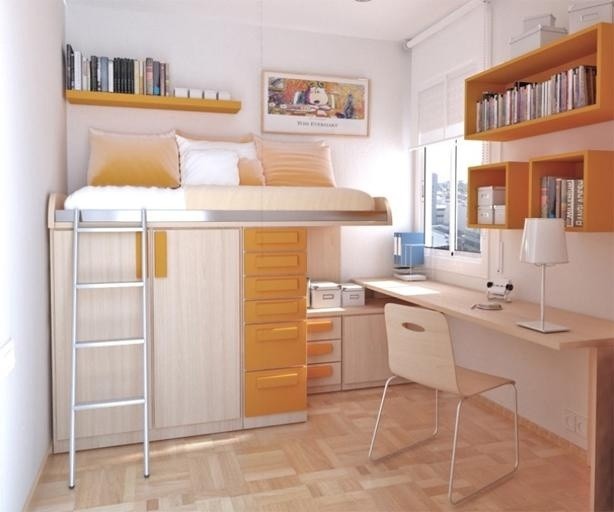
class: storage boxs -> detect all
[523,14,556,32]
[339,282,365,307]
[567,0,614,35]
[510,23,568,58]
[310,281,342,309]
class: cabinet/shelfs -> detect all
[528,149,614,232]
[464,22,614,141]
[467,161,528,229]
[307,316,342,394]
[49,226,308,453]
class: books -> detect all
[475,63,597,133]
[540,175,583,228]
[65,42,171,96]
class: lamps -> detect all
[517,217,571,333]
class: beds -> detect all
[47,186,392,228]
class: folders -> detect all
[393,232,425,266]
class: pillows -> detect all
[176,130,265,187]
[254,137,326,147]
[261,144,336,187]
[86,127,180,188]
[176,130,255,143]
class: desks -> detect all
[352,278,614,512]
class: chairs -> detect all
[367,302,519,506]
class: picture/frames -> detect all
[261,68,370,137]
